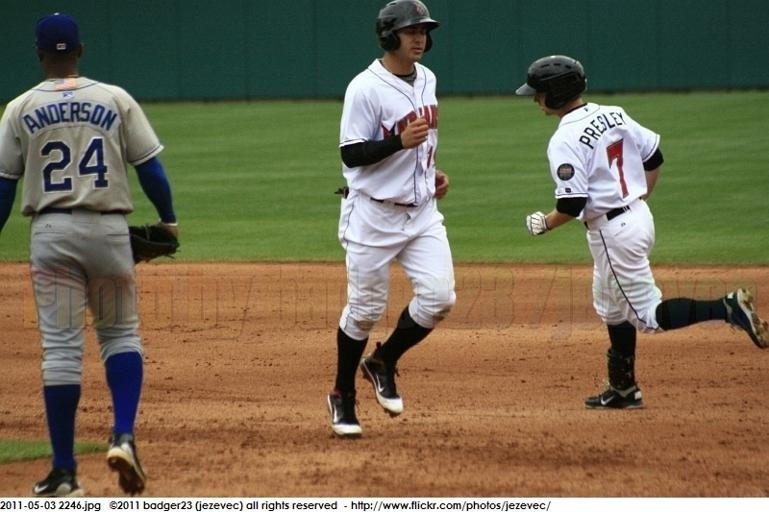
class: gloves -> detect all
[526,211,549,238]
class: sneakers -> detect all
[361,355,407,418]
[326,389,365,438]
[723,287,768,351]
[582,387,645,412]
[106,436,147,497]
[31,472,82,498]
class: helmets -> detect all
[516,56,586,111]
[376,0,440,51]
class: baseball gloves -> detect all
[129,225,178,264]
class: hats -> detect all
[34,11,80,52]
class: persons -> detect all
[322,1,462,441]
[512,52,768,412]
[0,11,183,497]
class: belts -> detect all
[584,195,643,230]
[38,208,124,215]
